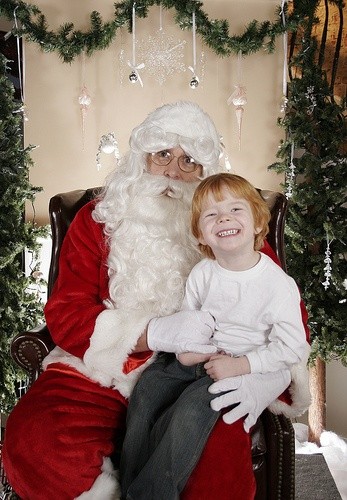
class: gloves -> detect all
[207,366,292,433]
[147,310,218,353]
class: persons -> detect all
[120,174,306,499]
[2,102,312,499]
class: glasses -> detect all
[149,149,200,172]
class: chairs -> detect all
[9,185,295,500]
